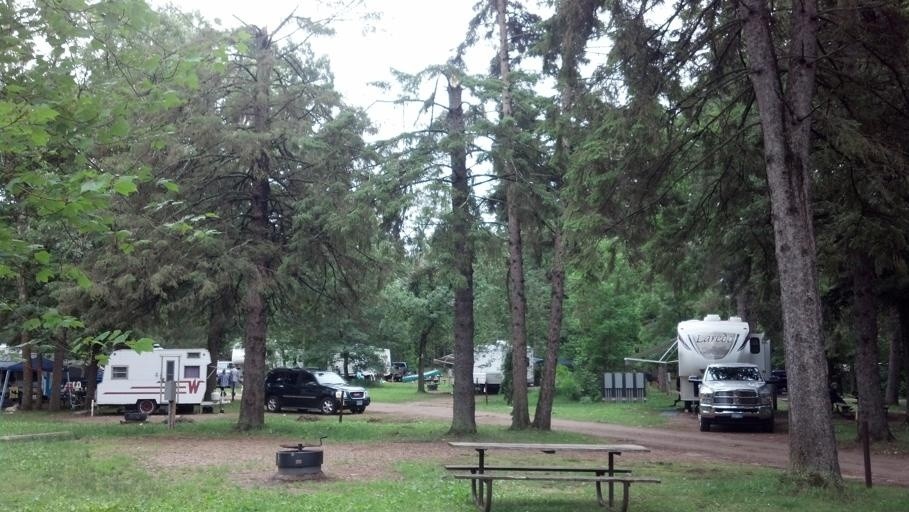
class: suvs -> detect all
[216,360,240,387]
[264,367,371,415]
[385,361,412,382]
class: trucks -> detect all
[448,360,506,394]
[687,363,780,433]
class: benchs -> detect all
[445,462,631,501]
[454,472,662,510]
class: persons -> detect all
[217,367,229,403]
[229,365,240,402]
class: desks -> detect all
[447,441,651,511]
